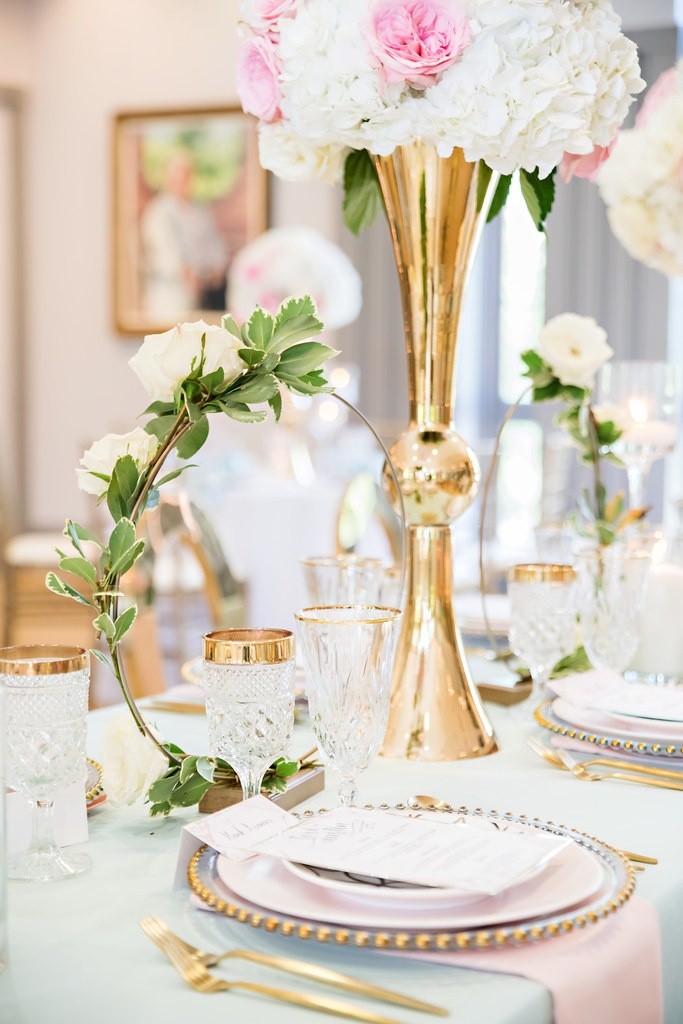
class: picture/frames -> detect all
[107,94,270,332]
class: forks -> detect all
[559,750,683,791]
[142,913,447,1015]
[526,736,682,779]
[154,936,402,1024]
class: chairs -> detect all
[178,491,250,629]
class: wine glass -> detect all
[201,628,296,801]
[1,645,90,880]
[509,563,579,722]
[294,605,403,809]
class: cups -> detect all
[305,556,381,605]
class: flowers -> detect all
[596,59,683,279]
[44,297,343,813]
[235,0,647,182]
[519,308,650,675]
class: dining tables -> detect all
[0,554,683,1024]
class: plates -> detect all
[278,847,488,911]
[85,757,104,802]
[553,696,682,742]
[188,804,635,948]
[534,698,683,757]
[213,811,605,930]
